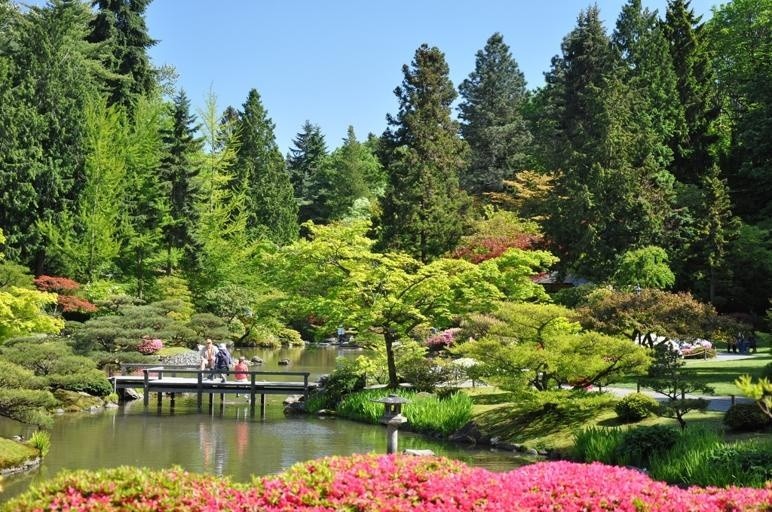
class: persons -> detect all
[199,339,220,381]
[202,342,234,382]
[233,356,249,399]
